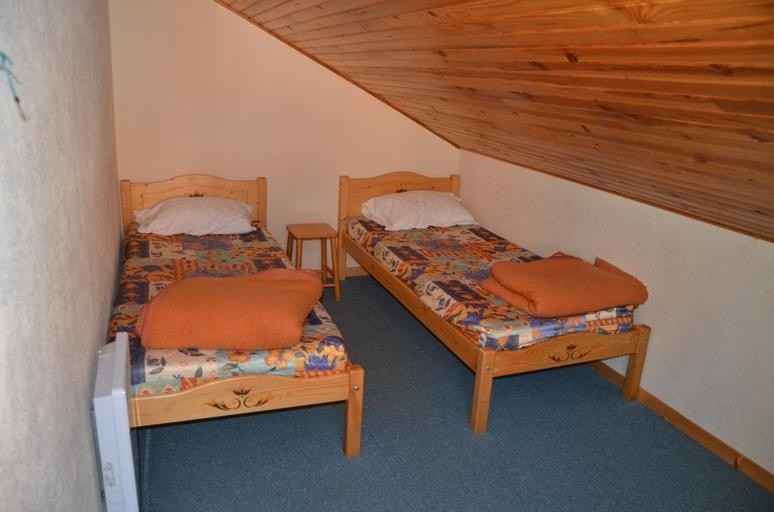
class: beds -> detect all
[103,170,366,458]
[333,168,654,433]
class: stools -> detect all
[284,222,341,303]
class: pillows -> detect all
[129,194,262,239]
[356,189,481,234]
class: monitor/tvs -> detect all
[93,331,142,512]
